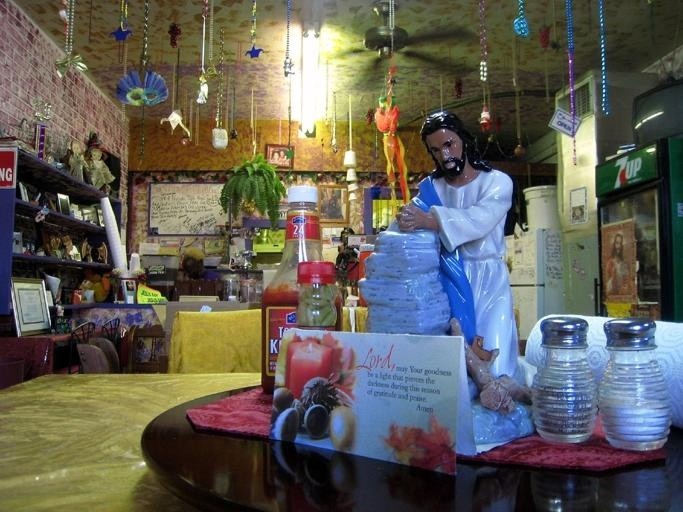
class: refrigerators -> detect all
[594,137,683,321]
[505,230,543,353]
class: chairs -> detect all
[88,337,120,374]
[54,322,96,374]
[167,308,263,373]
[76,344,110,374]
[99,317,120,343]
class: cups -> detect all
[99,195,142,274]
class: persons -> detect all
[271,149,288,162]
[396,110,526,385]
[66,139,117,191]
[604,232,633,296]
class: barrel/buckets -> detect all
[522,185,562,232]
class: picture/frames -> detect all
[266,144,295,169]
[10,276,52,338]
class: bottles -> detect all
[240,251,257,271]
[260,183,340,396]
[222,274,257,304]
[530,314,673,452]
[526,464,670,509]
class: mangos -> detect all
[329,405,354,450]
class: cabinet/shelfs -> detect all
[172,280,227,302]
[0,146,121,336]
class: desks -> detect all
[0,373,682,512]
